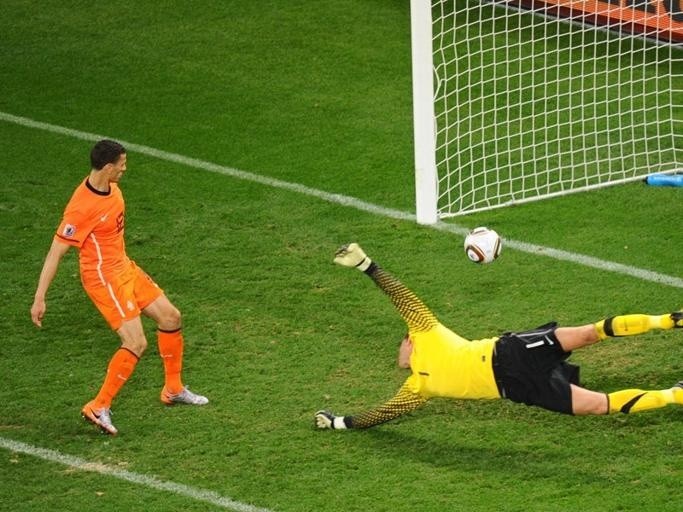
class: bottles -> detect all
[642,174,683,186]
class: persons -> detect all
[308,241,682,431]
[30,138,210,437]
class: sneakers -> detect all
[160,384,209,406]
[80,400,118,434]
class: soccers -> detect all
[464,227,503,264]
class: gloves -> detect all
[332,242,372,272]
[315,411,337,430]
